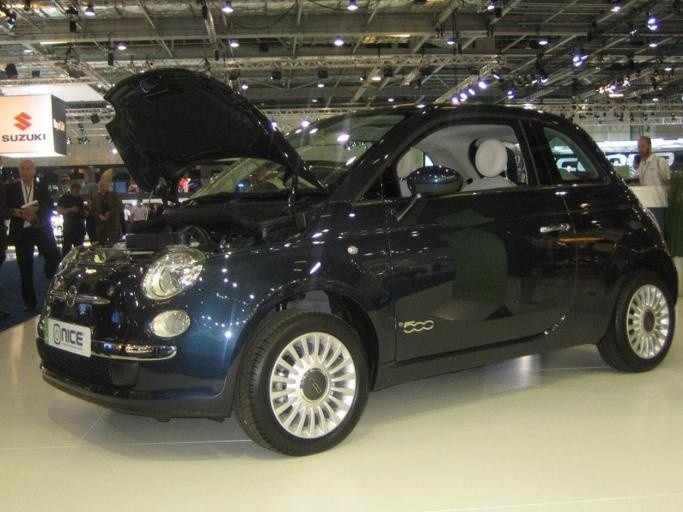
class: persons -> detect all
[96,182,123,243]
[124,204,131,222]
[131,199,146,224]
[633,155,641,174]
[57,184,86,256]
[637,136,671,239]
[0,160,59,312]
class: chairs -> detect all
[462,140,518,191]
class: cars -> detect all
[35,66,679,458]
